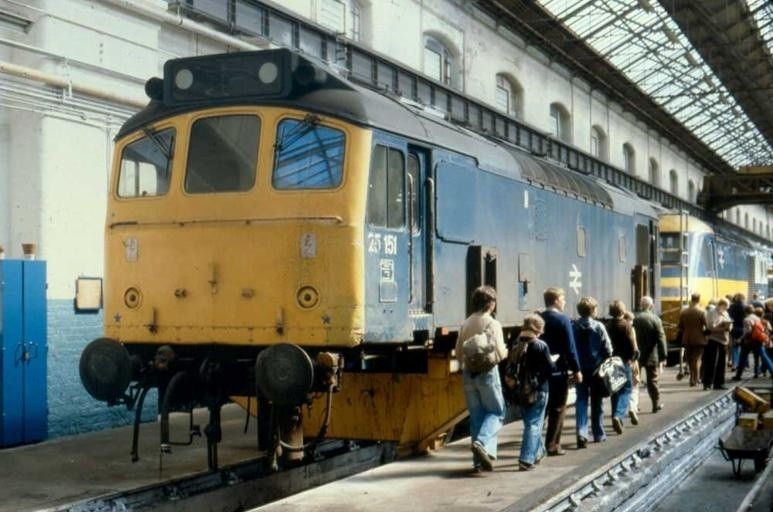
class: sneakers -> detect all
[469,404,664,473]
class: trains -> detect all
[78,43,772,480]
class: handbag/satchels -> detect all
[462,330,500,379]
[592,356,628,397]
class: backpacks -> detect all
[752,319,769,345]
[502,338,545,408]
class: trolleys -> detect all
[712,382,771,479]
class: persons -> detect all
[572,297,614,449]
[538,287,584,456]
[455,285,509,474]
[624,313,639,426]
[604,300,633,434]
[633,296,668,412]
[675,291,773,392]
[499,314,554,472]
